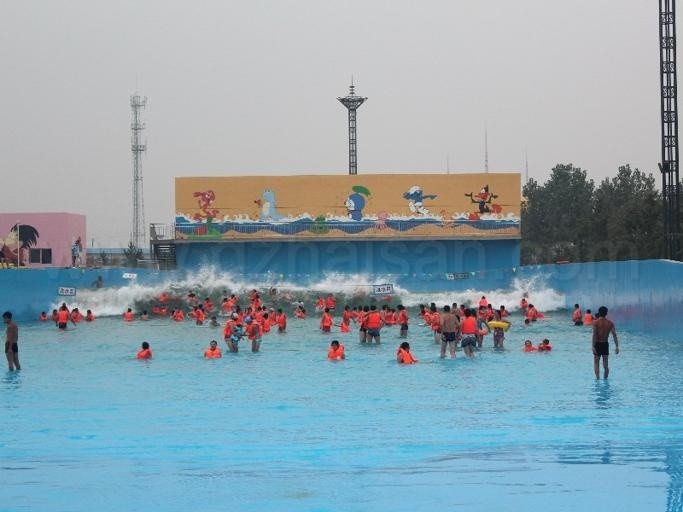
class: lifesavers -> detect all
[478,321,508,335]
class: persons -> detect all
[3,312,21,370]
[39,288,599,365]
[593,306,619,379]
